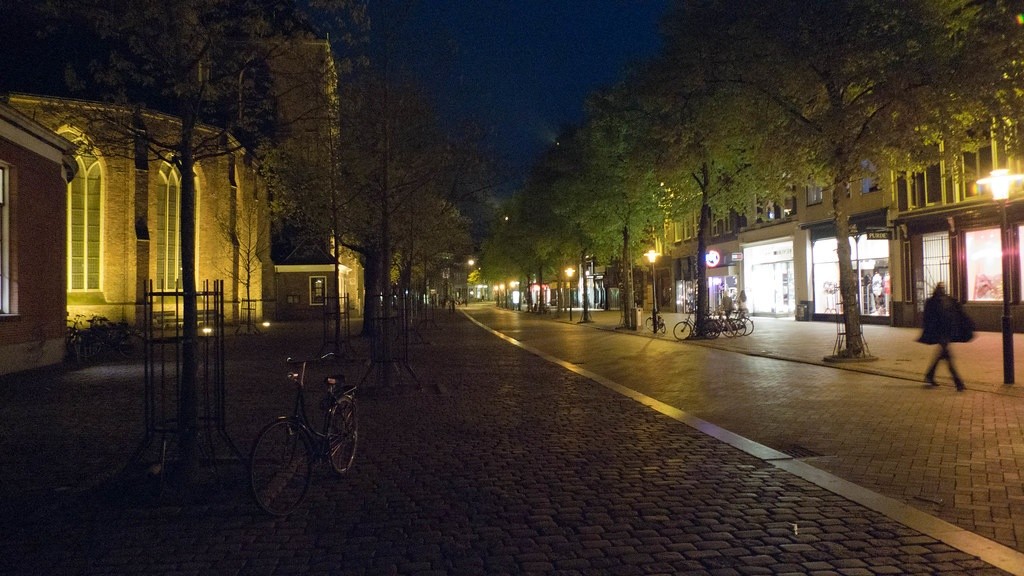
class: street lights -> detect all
[564,268,575,321]
[643,249,661,334]
[975,167,1024,384]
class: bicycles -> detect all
[247,352,360,518]
[62,314,145,359]
[646,308,666,334]
[673,308,755,340]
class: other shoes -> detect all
[925,376,939,387]
[954,378,966,391]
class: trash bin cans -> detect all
[630,306,644,330]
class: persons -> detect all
[866,271,890,296]
[723,292,732,310]
[736,290,747,309]
[914,282,977,392]
